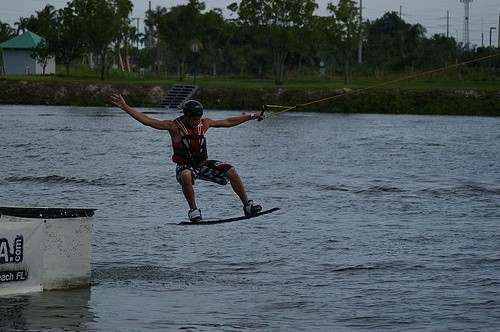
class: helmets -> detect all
[184,101,203,116]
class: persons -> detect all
[110,91,265,222]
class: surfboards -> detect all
[178,207,280,225]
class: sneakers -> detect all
[243,200,261,214]
[188,209,202,220]
